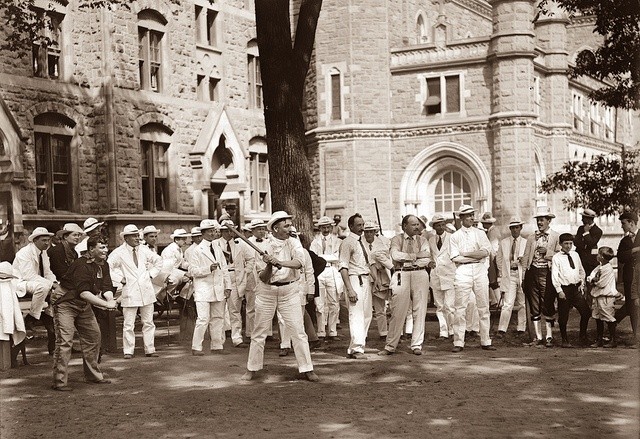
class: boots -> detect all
[589,319,604,348]
[560,324,572,348]
[579,322,593,345]
[603,322,617,348]
[10,338,23,369]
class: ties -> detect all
[437,236,442,250]
[406,238,414,255]
[39,251,43,278]
[512,238,516,261]
[564,252,575,269]
[226,241,233,264]
[369,244,372,252]
[209,243,216,261]
[465,229,473,252]
[357,236,369,264]
[133,248,138,268]
[322,237,326,253]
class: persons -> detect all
[361,222,389,340]
[418,214,434,240]
[160,229,194,306]
[377,206,431,356]
[479,211,502,310]
[141,224,166,307]
[51,234,118,391]
[496,215,527,339]
[107,224,162,355]
[338,214,373,360]
[464,290,480,337]
[10,224,54,325]
[75,215,108,257]
[322,286,343,330]
[449,205,493,352]
[310,216,344,343]
[400,298,412,341]
[428,214,457,340]
[216,220,255,347]
[611,212,640,342]
[47,222,83,281]
[587,247,618,347]
[241,212,319,381]
[551,232,591,346]
[575,209,603,274]
[182,219,229,356]
[522,207,557,346]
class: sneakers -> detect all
[71,346,81,354]
[49,350,54,355]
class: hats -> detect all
[314,216,335,227]
[479,212,496,224]
[141,225,161,239]
[82,217,104,233]
[247,219,270,229]
[240,223,252,232]
[56,222,87,238]
[195,219,218,231]
[454,205,478,216]
[429,214,449,227]
[267,211,296,232]
[363,221,380,231]
[333,215,341,221]
[532,206,556,218]
[169,228,190,240]
[579,209,598,217]
[218,220,238,229]
[120,224,142,241]
[289,225,301,235]
[211,219,221,230]
[190,226,202,236]
[506,216,525,227]
[416,215,428,229]
[28,227,55,242]
[474,215,481,223]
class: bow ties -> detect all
[535,231,550,242]
[148,245,154,249]
[256,238,263,242]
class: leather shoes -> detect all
[51,383,73,391]
[377,349,394,355]
[436,336,448,341]
[464,330,470,340]
[123,354,134,359]
[328,336,341,341]
[482,345,497,351]
[226,330,231,337]
[349,352,367,359]
[236,343,249,348]
[451,334,454,342]
[40,314,53,333]
[278,348,290,356]
[528,337,543,346]
[319,337,326,341]
[23,315,37,332]
[211,349,231,355]
[245,337,251,344]
[240,369,255,381]
[413,348,422,355]
[451,346,463,353]
[266,336,277,342]
[146,353,159,357]
[470,331,478,337]
[545,337,554,346]
[400,335,406,340]
[496,331,504,339]
[406,333,412,340]
[380,336,386,339]
[305,371,319,382]
[174,296,187,306]
[518,331,525,339]
[94,379,112,384]
[309,340,321,351]
[192,350,204,356]
[336,324,342,329]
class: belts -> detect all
[268,277,301,286]
[227,269,234,272]
[395,267,425,271]
[510,266,518,270]
[563,283,581,287]
[348,273,369,285]
[460,262,481,264]
